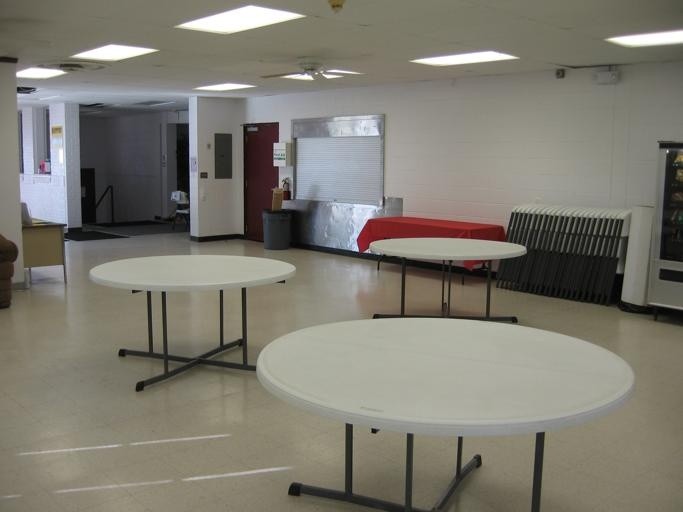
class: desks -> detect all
[89,254,296,391]
[256,318,635,512]
[358,217,505,285]
[369,238,527,322]
[23,219,69,284]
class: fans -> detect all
[261,63,362,80]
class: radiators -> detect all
[494,204,633,308]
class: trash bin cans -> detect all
[262,209,291,250]
